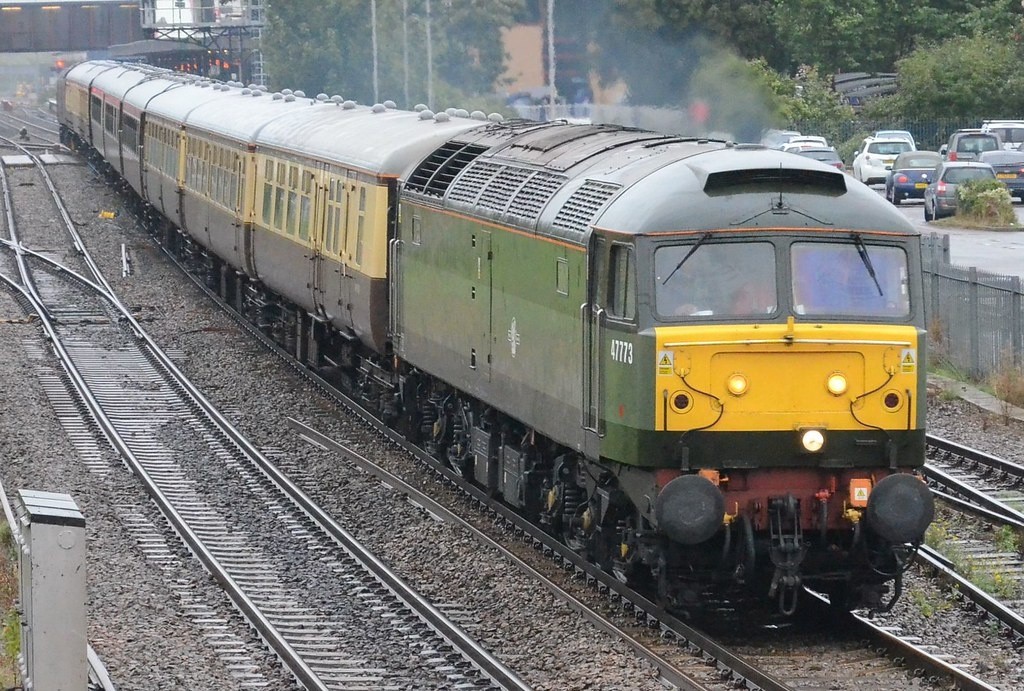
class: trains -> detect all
[52,34,935,639]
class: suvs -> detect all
[850,136,914,187]
[981,117,1024,150]
[945,128,1004,163]
[921,161,996,222]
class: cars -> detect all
[873,129,920,149]
[938,143,947,159]
[885,150,944,205]
[766,129,847,176]
[974,150,1024,205]
